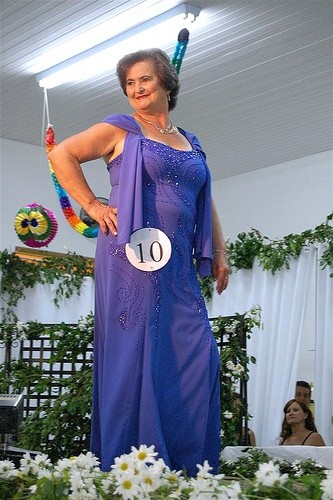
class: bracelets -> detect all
[212,250,227,256]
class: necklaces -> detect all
[144,119,174,134]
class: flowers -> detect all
[0,444,325,500]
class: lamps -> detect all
[33,2,202,89]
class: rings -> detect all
[225,277,228,280]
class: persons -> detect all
[224,392,256,447]
[48,49,230,479]
[277,380,326,446]
